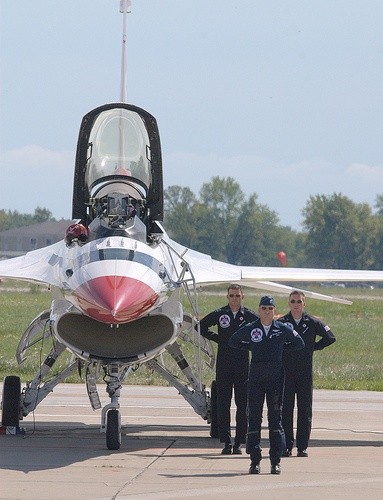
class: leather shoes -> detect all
[282,448,293,457]
[270,463,281,473]
[249,461,261,474]
[222,445,232,454]
[233,446,242,454]
[296,449,309,456]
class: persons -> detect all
[194,283,260,455]
[277,290,336,457]
[229,296,305,474]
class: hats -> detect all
[259,295,275,307]
[65,223,89,238]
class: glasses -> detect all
[261,307,273,311]
[290,300,302,304]
[228,294,241,298]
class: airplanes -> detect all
[0,95,383,453]
